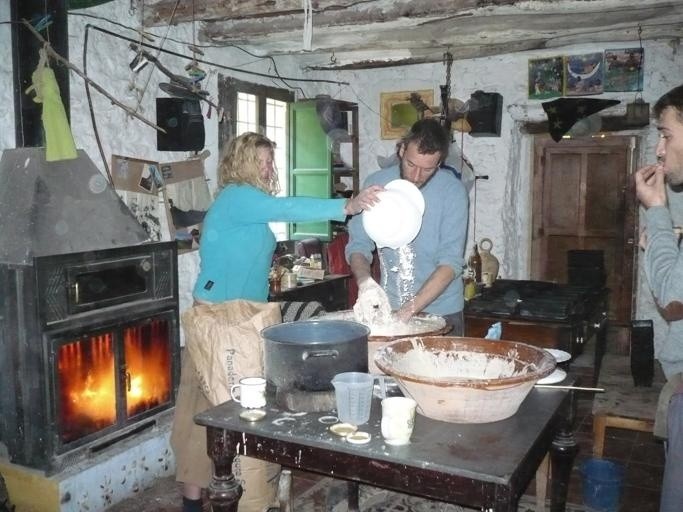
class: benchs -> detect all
[591,325,667,460]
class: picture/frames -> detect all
[381,88,434,140]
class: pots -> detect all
[258,317,371,414]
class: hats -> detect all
[159,75,206,101]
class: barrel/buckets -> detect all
[580,458,627,512]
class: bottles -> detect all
[469,240,481,281]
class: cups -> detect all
[267,274,281,296]
[283,271,298,291]
[310,253,323,270]
[380,395,417,446]
[229,376,268,409]
[329,370,386,428]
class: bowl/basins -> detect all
[328,305,452,371]
[362,179,425,251]
[376,337,556,425]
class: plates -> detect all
[537,365,567,385]
[297,278,316,284]
[545,347,572,363]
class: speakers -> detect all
[156,98,205,151]
[468,91,503,136]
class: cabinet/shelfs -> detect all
[297,96,360,236]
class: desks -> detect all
[269,273,351,312]
[195,371,584,512]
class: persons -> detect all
[342,116,469,337]
[632,82,682,380]
[169,132,386,512]
